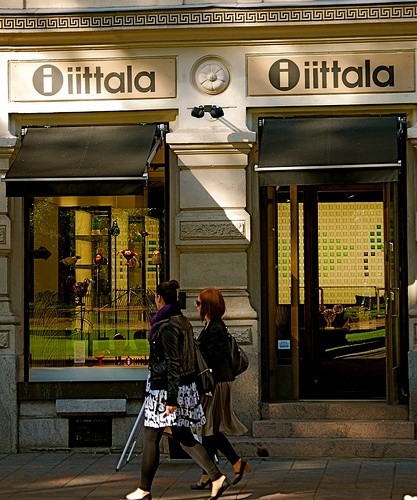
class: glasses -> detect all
[196,300,202,306]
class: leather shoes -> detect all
[208,476,232,500]
[119,492,152,500]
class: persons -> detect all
[189,287,251,490]
[120,279,232,500]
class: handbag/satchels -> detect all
[194,340,214,394]
[226,332,249,376]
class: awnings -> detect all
[0,125,162,179]
[256,112,406,187]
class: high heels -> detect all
[190,478,212,489]
[232,458,251,485]
